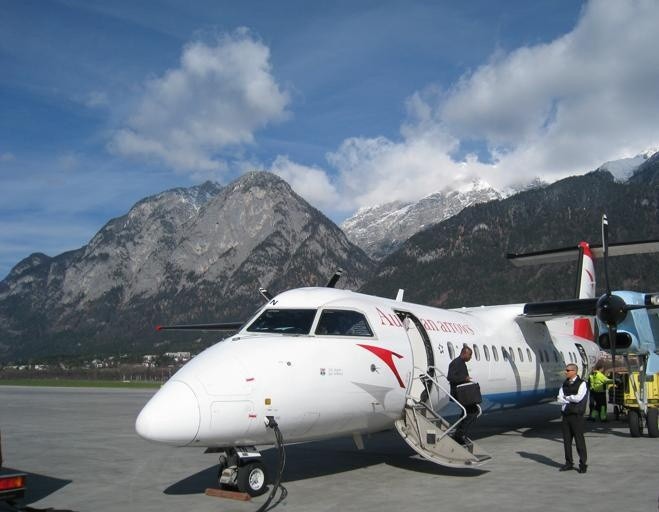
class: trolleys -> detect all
[605,370,639,420]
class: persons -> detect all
[557,364,588,473]
[589,365,613,423]
[446,346,479,445]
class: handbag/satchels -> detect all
[456,382,482,405]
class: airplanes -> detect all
[134,213,659,495]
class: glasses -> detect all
[566,369,575,372]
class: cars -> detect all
[0,465,25,507]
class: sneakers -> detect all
[560,466,574,471]
[578,467,587,474]
